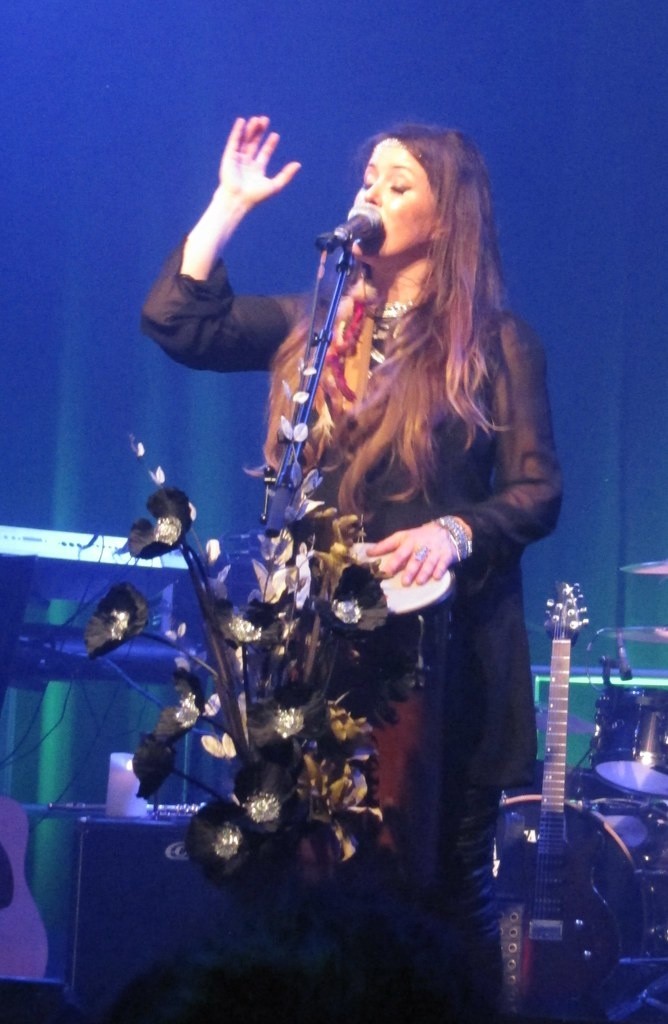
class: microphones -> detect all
[314,205,381,254]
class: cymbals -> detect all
[598,627,667,644]
[618,562,668,575]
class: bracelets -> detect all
[429,513,473,565]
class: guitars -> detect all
[499,584,625,1022]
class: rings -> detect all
[412,547,432,561]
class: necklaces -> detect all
[371,298,414,314]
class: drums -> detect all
[593,686,668,798]
[492,796,655,1024]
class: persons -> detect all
[140,115,566,1024]
